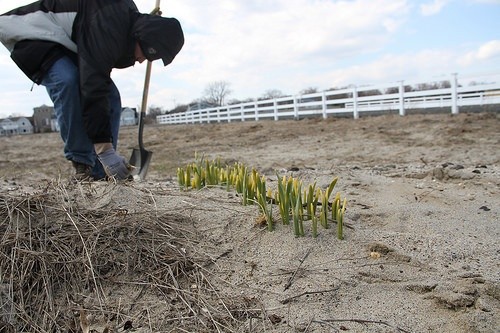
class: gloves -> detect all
[97,148,131,180]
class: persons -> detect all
[0,1,185,181]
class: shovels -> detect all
[124,0,161,183]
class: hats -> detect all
[139,38,162,62]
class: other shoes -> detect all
[73,162,89,179]
